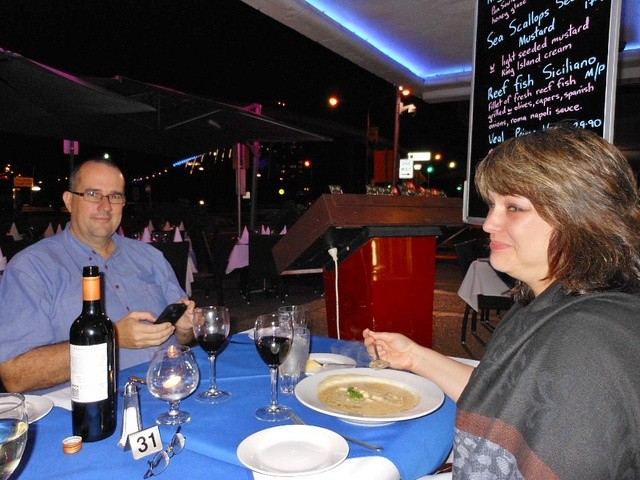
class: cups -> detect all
[273,326,312,396]
[276,304,311,330]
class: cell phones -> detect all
[152,302,187,326]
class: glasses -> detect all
[143,425,186,479]
[67,189,126,205]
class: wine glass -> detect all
[252,312,295,422]
[193,306,232,407]
[0,391,30,479]
[146,346,200,426]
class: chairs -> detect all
[146,240,191,293]
[454,240,514,346]
[196,227,248,305]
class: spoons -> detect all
[369,342,391,370]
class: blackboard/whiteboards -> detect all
[462,0,622,225]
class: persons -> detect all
[0,156,206,395]
[360,124,640,479]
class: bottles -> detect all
[115,383,143,449]
[69,265,119,445]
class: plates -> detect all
[294,367,446,427]
[304,351,358,377]
[0,393,54,425]
[236,423,350,477]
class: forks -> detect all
[285,408,384,453]
